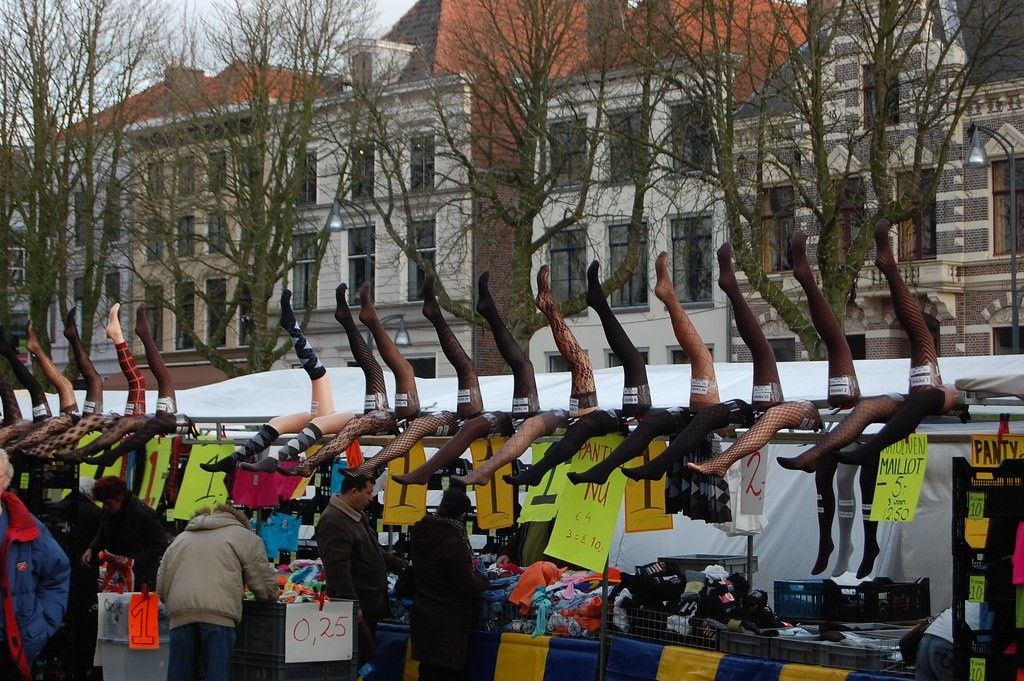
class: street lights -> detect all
[963,123,1020,355]
[366,312,412,354]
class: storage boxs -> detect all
[774,576,930,622]
[228,599,360,681]
[656,553,757,576]
[720,624,918,672]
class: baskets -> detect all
[657,553,929,672]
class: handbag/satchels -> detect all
[97,548,136,595]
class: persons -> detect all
[497,516,593,570]
[0,447,167,680]
[0,303,192,467]
[407,486,489,681]
[916,606,953,681]
[314,474,412,681]
[199,218,957,486]
[155,505,281,681]
[811,441,881,579]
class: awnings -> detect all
[102,363,254,390]
